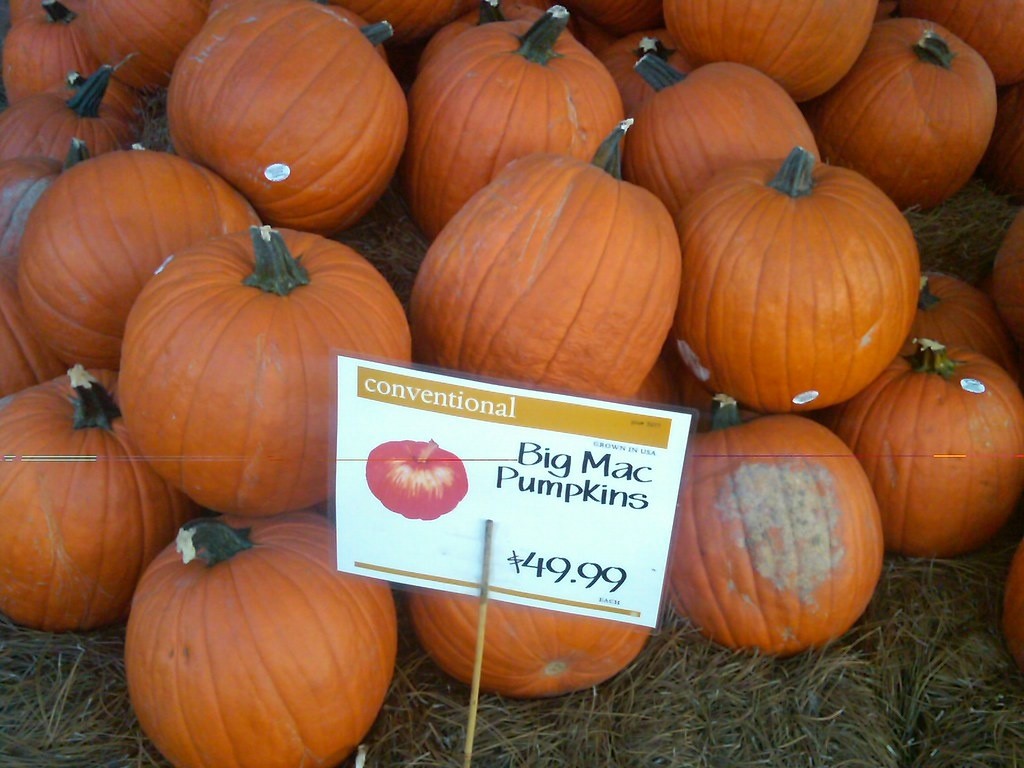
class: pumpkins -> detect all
[0,0,1024,768]
